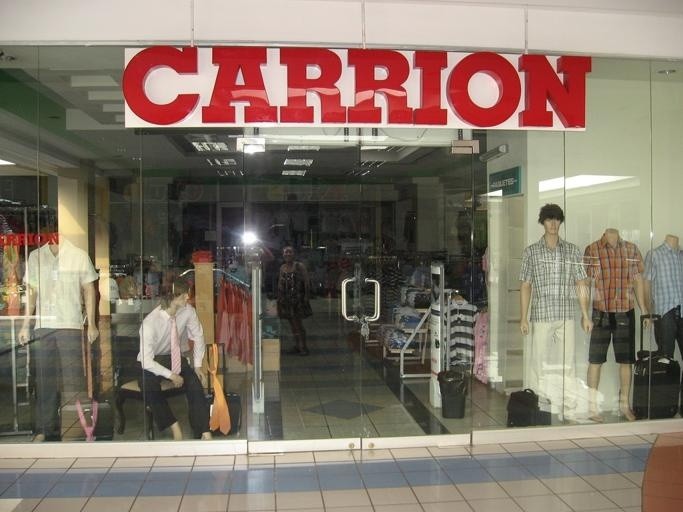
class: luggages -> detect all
[202,343,243,436]
[57,397,114,441]
[632,312,680,419]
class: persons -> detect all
[642,234,683,418]
[256,241,275,266]
[17,232,100,442]
[136,276,215,440]
[276,244,313,357]
[519,202,591,416]
[581,228,651,421]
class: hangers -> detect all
[451,293,468,304]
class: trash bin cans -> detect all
[437,372,466,418]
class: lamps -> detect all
[477,143,508,164]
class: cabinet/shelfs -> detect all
[381,256,435,378]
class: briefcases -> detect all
[506,387,553,426]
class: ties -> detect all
[206,343,233,435]
[74,399,99,441]
[169,316,183,377]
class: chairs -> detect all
[111,337,197,440]
